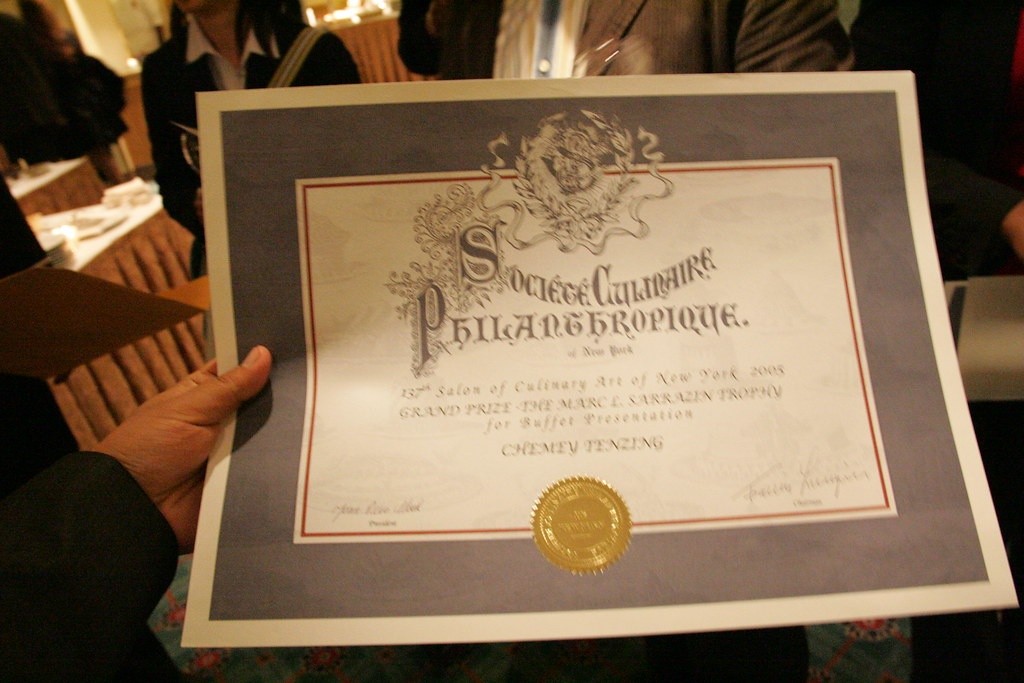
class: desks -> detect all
[22,204,209,453]
[296,0,422,83]
[0,158,106,216]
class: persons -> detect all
[139,0,362,357]
[395,0,1024,683]
[1,0,112,179]
[1,169,271,683]
